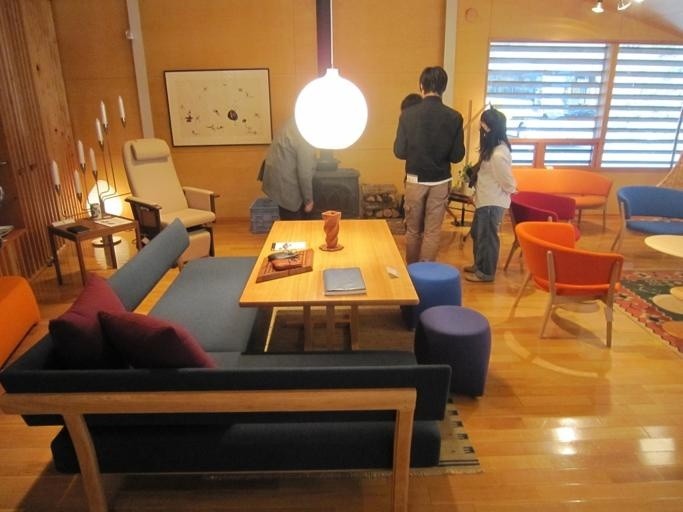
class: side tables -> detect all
[46,210,142,287]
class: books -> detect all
[323,268,366,296]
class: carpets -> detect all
[603,268,683,357]
[55,304,485,482]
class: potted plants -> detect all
[459,161,475,197]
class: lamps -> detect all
[617,0,632,11]
[591,0,607,14]
[93,240,129,269]
[85,177,123,249]
[293,69,370,151]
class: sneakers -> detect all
[464,265,478,273]
[465,274,485,282]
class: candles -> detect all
[73,168,81,195]
[100,99,108,126]
[78,139,85,165]
[88,146,97,173]
[95,118,104,142]
[51,160,61,186]
[117,94,126,120]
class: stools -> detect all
[414,307,493,401]
[400,261,463,331]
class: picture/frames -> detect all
[162,68,274,149]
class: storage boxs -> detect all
[249,196,279,234]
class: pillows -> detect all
[46,273,126,370]
[96,308,217,369]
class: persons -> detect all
[464,107,517,282]
[259,114,314,219]
[394,66,466,265]
[399,93,422,109]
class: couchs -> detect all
[1,218,454,491]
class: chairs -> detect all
[510,168,613,231]
[502,190,581,276]
[611,186,683,254]
[123,137,220,264]
[507,223,624,347]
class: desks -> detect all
[645,233,683,315]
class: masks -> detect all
[479,128,489,141]
